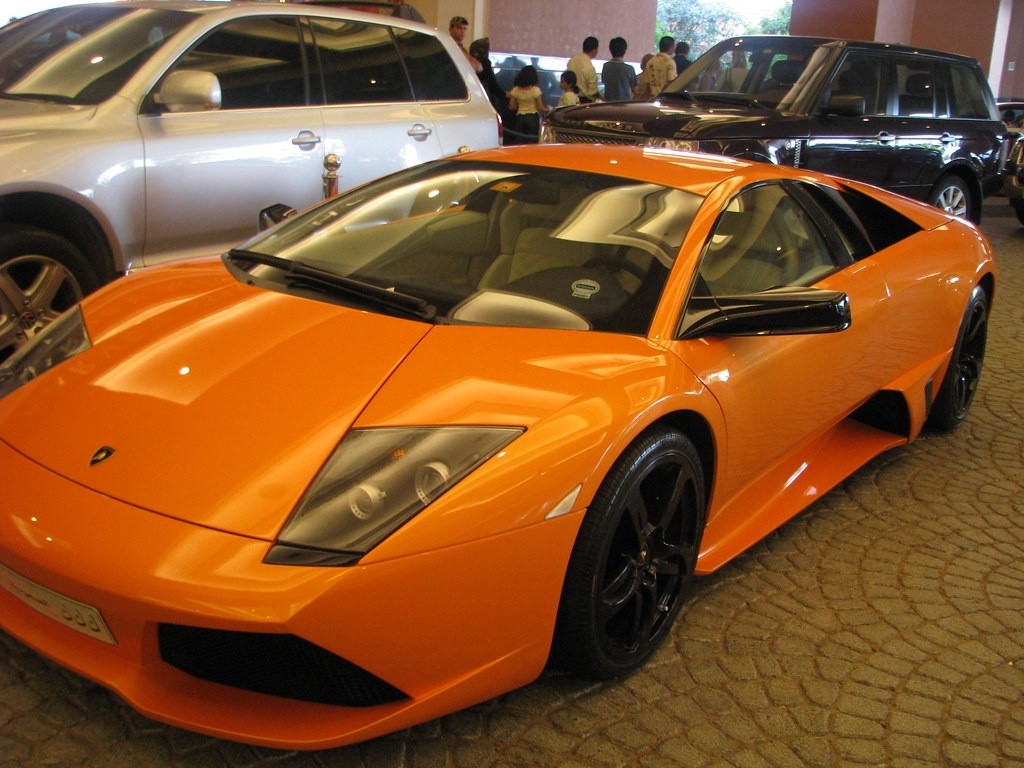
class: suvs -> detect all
[0,0,504,370]
[538,34,1008,235]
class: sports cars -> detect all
[0,144,996,752]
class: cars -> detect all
[995,102,1024,225]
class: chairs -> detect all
[477,201,623,293]
[759,61,804,92]
[708,211,785,295]
[830,71,886,115]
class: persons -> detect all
[449,16,515,110]
[508,66,551,145]
[493,55,557,94]
[1001,110,1015,124]
[555,35,750,111]
